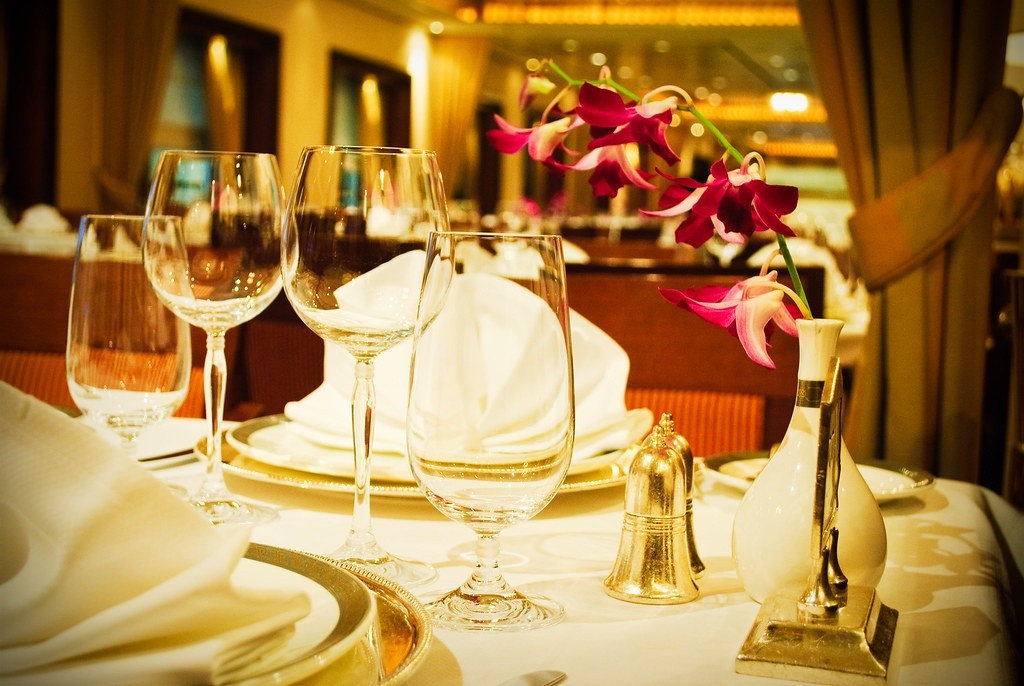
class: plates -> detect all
[704,453,935,504]
[226,413,626,485]
[232,541,375,686]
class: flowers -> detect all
[485,58,815,369]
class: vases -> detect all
[731,319,887,606]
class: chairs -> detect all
[625,389,765,457]
[0,351,204,418]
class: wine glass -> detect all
[63,212,190,452]
[282,143,454,592]
[407,231,576,632]
[143,150,300,524]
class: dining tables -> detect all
[145,459,1007,685]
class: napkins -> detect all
[0,379,310,686]
[284,249,654,474]
